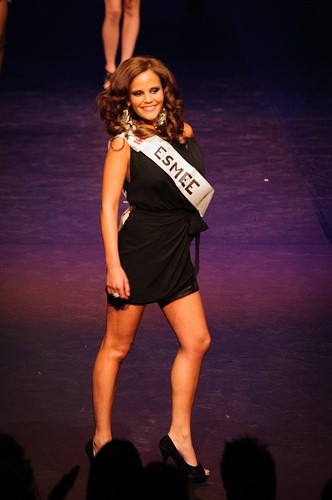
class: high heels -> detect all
[158,434,209,481]
[103,69,116,90]
[86,437,95,462]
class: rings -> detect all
[114,293,119,298]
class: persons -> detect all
[0,432,332,500]
[102,0,140,91]
[92,55,212,484]
